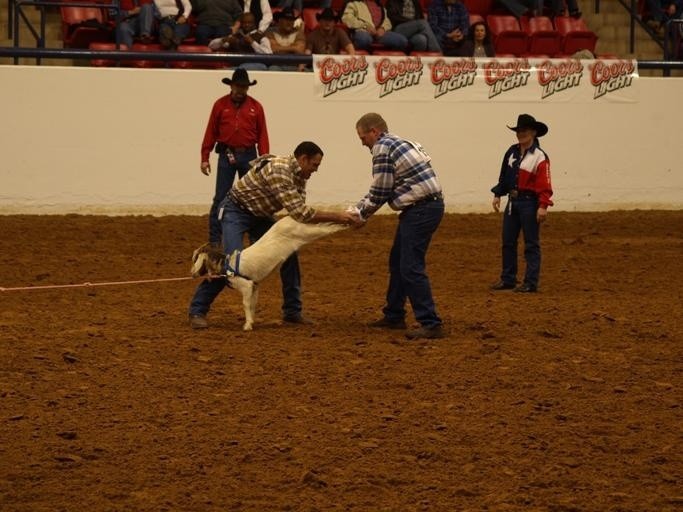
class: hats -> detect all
[221,68,257,86]
[506,114,548,137]
[315,8,339,23]
[273,7,301,22]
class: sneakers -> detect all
[282,316,315,327]
[366,318,408,329]
[405,324,445,339]
[188,312,209,329]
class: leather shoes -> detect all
[513,280,537,293]
[492,280,516,290]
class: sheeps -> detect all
[189,212,354,331]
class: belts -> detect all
[508,194,538,201]
[402,194,442,213]
[226,192,254,216]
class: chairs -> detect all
[57,0,599,71]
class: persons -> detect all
[185,139,365,329]
[198,68,273,249]
[345,112,446,338]
[115,1,494,68]
[487,113,555,295]
